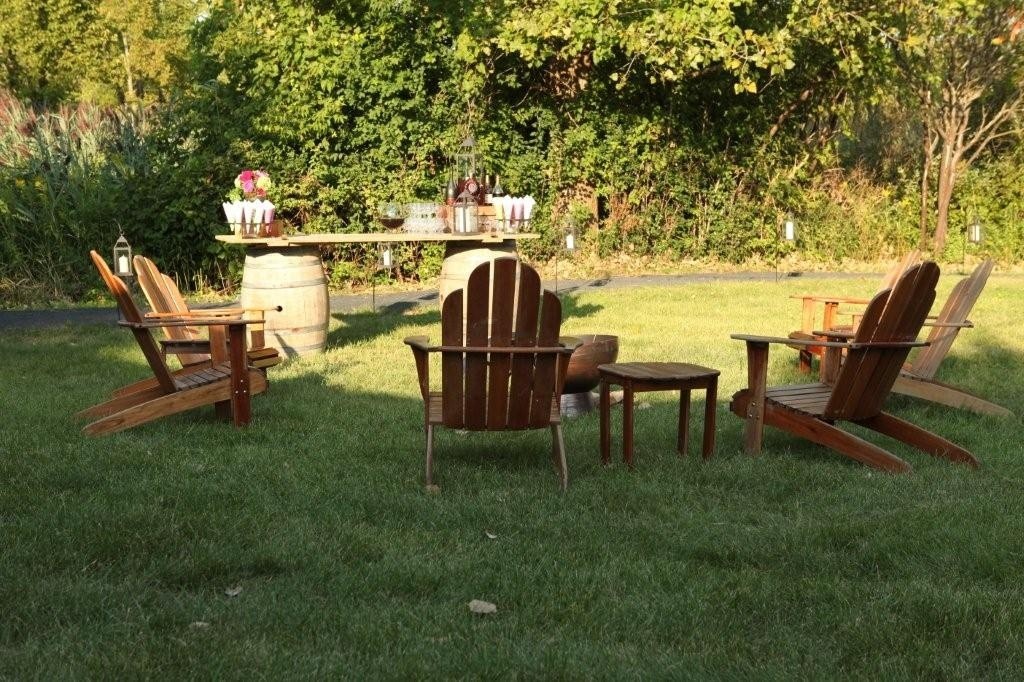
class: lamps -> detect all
[113,234,134,276]
[451,187,480,236]
[377,241,399,269]
[968,216,979,243]
[562,222,575,251]
[782,214,793,239]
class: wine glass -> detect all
[404,202,447,234]
[378,201,406,234]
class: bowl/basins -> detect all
[557,334,619,394]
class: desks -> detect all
[214,233,540,359]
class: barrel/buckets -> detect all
[240,248,331,359]
[439,239,522,357]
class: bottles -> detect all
[441,164,504,205]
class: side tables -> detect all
[596,362,720,464]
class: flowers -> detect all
[225,170,274,200]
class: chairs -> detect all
[77,249,282,437]
[402,258,585,490]
[787,250,1014,416]
[728,262,978,473]
